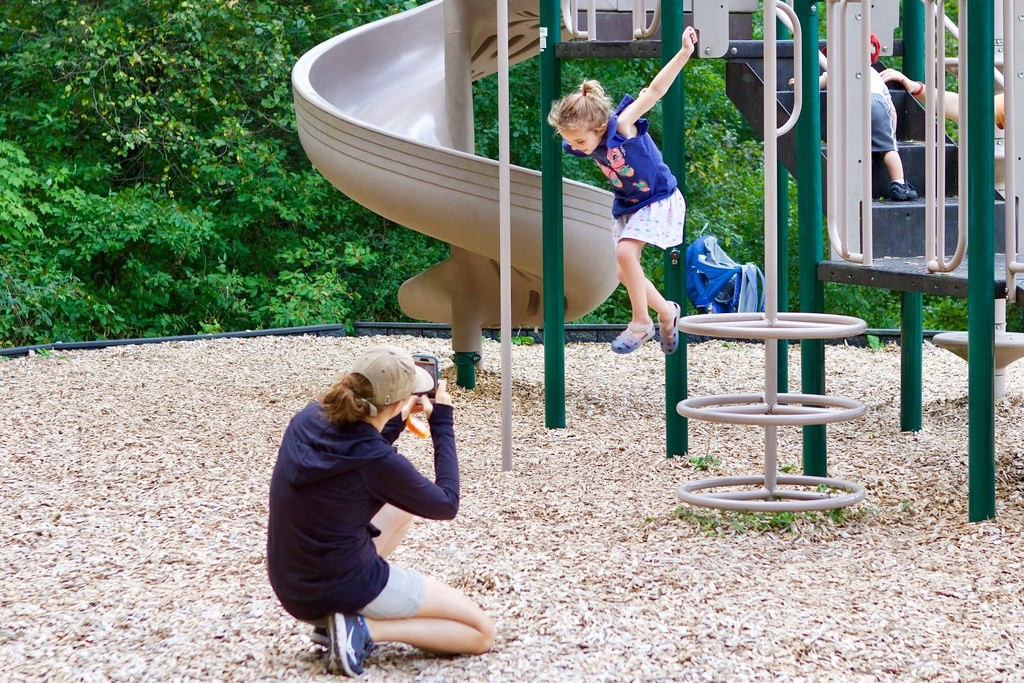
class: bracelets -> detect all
[912,81,923,96]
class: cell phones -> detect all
[411,355,438,400]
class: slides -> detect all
[286,1,636,330]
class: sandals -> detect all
[657,301,681,354]
[611,317,656,354]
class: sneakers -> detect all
[889,180,918,201]
[329,612,366,678]
[310,626,373,656]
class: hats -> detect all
[349,346,435,405]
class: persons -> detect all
[545,24,698,357]
[788,65,918,201]
[820,31,881,66]
[265,343,507,678]
[877,68,1005,131]
